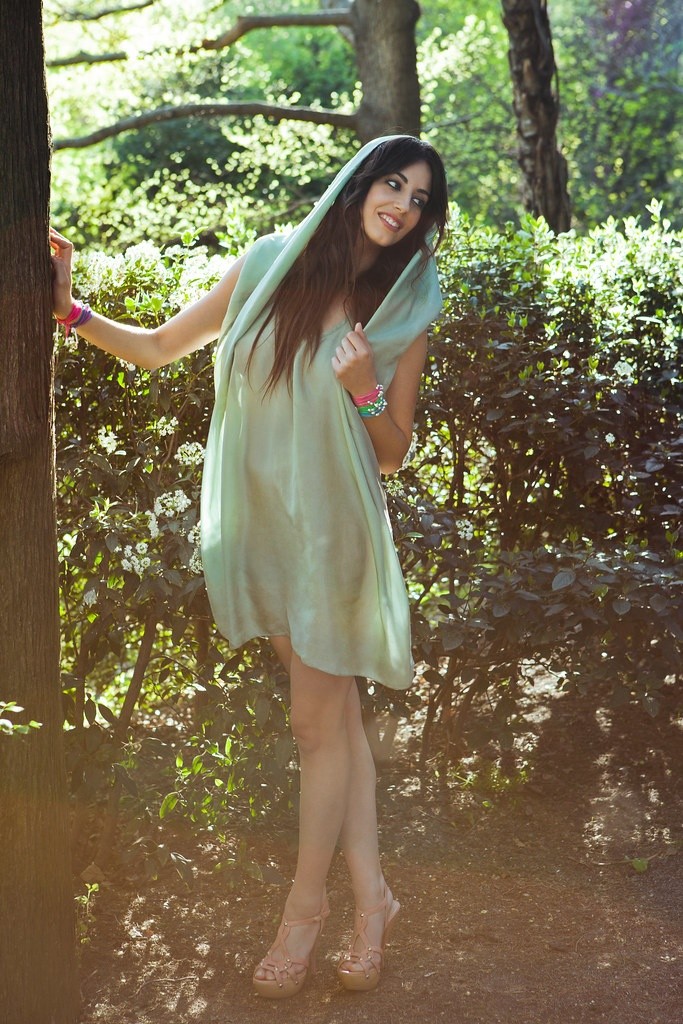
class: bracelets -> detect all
[353,384,387,417]
[55,300,93,338]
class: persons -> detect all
[49,135,450,998]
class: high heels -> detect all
[337,881,400,991]
[254,887,331,998]
